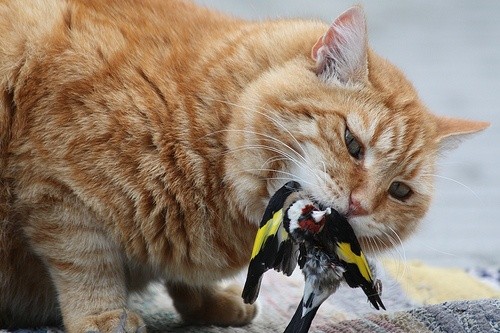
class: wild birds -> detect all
[242,180,387,333]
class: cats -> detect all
[0,0,491,333]
